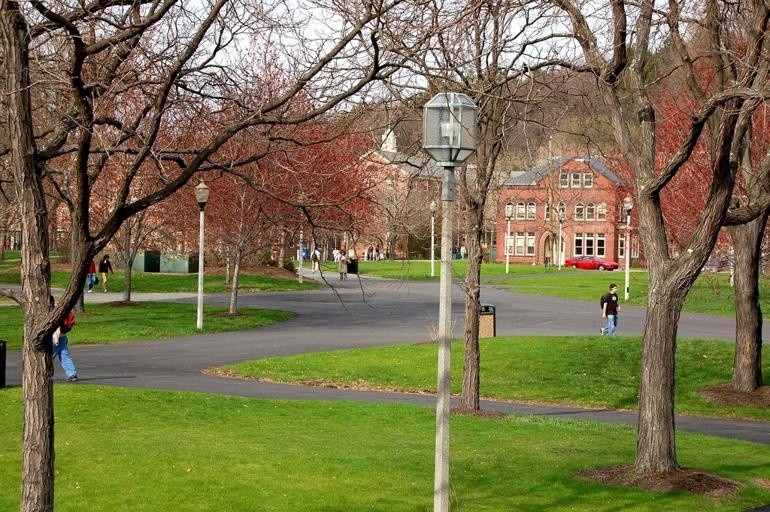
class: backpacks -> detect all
[601,293,618,309]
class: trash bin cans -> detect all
[347,258,358,274]
[478,303,496,338]
[0,339,8,389]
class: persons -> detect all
[86,259,98,293]
[453,246,458,259]
[339,251,351,281]
[365,243,388,260]
[99,254,114,293]
[460,243,466,260]
[301,245,310,266]
[50,296,79,382]
[310,247,321,274]
[600,283,620,336]
[331,245,355,263]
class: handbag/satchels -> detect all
[60,312,75,334]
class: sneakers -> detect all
[63,376,78,382]
[600,328,605,336]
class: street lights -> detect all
[427,199,439,278]
[505,207,514,275]
[191,175,212,332]
[419,88,484,512]
[623,192,636,301]
[557,209,564,272]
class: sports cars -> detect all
[564,254,619,272]
